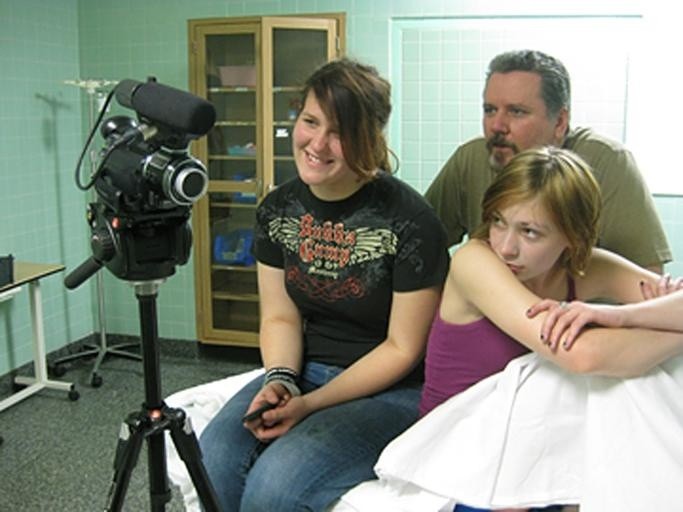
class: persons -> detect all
[421,47,674,289]
[415,144,682,510]
[194,57,451,511]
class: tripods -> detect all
[102,299,224,512]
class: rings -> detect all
[559,300,568,309]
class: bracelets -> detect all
[267,378,301,397]
[261,365,302,388]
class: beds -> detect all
[159,368,682,512]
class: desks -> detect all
[0,261,80,445]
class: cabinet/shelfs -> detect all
[187,13,346,358]
